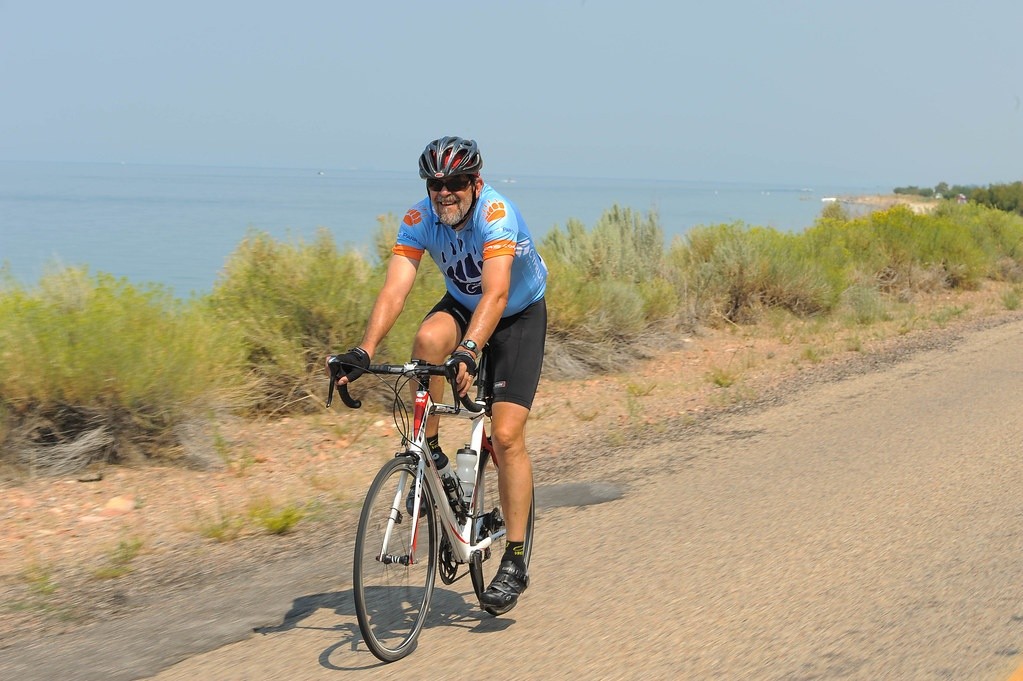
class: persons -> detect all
[325,136,550,607]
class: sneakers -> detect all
[406,454,451,518]
[479,554,530,607]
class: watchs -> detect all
[459,340,479,358]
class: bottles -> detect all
[432,452,464,502]
[455,443,477,503]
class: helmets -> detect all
[418,136,483,178]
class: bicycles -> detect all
[325,357,536,663]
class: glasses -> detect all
[427,176,470,191]
